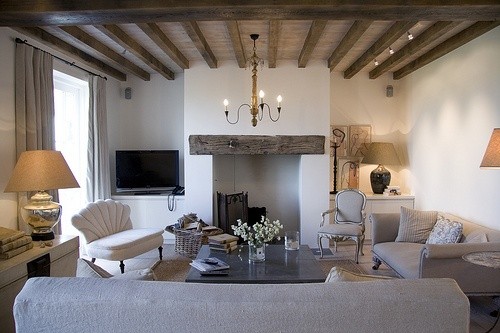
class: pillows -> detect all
[110,268,156,281]
[324,266,399,282]
[75,258,112,279]
[395,205,488,245]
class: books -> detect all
[189,256,231,275]
[0,227,33,260]
[208,234,239,253]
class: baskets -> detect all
[174,225,225,259]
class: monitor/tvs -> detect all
[115,150,179,196]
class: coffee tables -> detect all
[183,244,328,282]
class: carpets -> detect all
[149,256,367,282]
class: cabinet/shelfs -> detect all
[111,194,186,244]
[0,234,79,333]
[330,194,415,245]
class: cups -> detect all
[284,232,300,251]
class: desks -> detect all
[462,251,500,333]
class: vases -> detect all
[248,241,266,262]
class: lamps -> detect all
[480,127,500,169]
[374,31,414,65]
[4,150,81,241]
[223,33,282,127]
[361,142,399,194]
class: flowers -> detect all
[231,214,284,252]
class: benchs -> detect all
[367,210,500,317]
[71,199,164,274]
[13,277,470,333]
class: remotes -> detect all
[200,259,218,264]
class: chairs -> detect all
[316,189,367,264]
